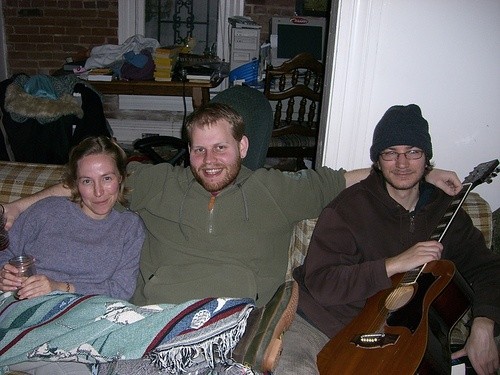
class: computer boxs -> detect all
[229,28,260,72]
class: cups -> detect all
[0,204,10,251]
[10,255,37,300]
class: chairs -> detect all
[260,54,325,172]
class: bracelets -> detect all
[65,281,71,295]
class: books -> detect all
[88,67,112,82]
[153,45,181,83]
[187,66,216,83]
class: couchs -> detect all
[0,162,493,375]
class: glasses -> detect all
[380,150,424,161]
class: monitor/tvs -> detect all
[270,16,326,72]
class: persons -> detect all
[272,103,499,375]
[0,135,159,375]
[0,102,465,375]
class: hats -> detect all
[370,104,433,164]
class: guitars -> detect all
[315,158,500,375]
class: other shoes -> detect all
[244,281,299,372]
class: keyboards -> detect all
[228,16,262,29]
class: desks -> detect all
[54,61,223,113]
